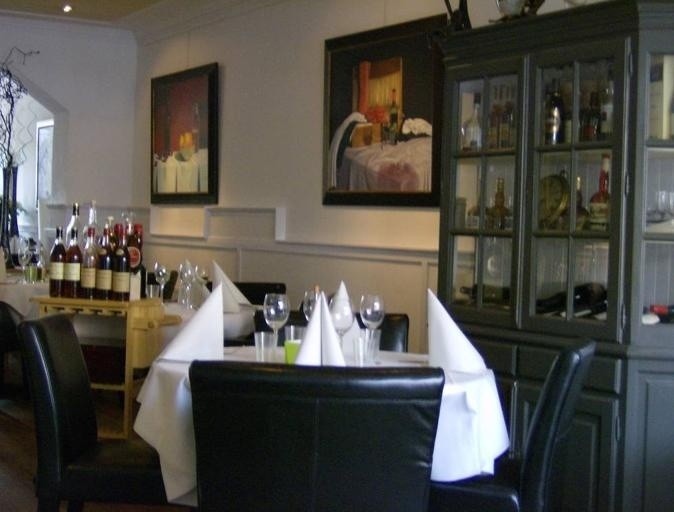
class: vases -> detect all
[371,124,381,146]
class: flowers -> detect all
[365,104,392,123]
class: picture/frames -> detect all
[150,62,219,204]
[322,13,449,206]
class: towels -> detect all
[157,260,255,362]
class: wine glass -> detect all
[10,233,46,286]
[327,298,354,363]
[261,293,289,358]
[152,259,210,312]
[359,294,384,363]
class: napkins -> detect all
[156,282,224,362]
[211,259,256,314]
[170,258,211,308]
[294,284,358,368]
[427,288,486,374]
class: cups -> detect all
[302,289,325,321]
[283,325,307,363]
[654,191,673,224]
[253,331,278,361]
[145,285,158,298]
[351,327,382,362]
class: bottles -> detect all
[388,89,397,145]
[163,107,171,158]
[573,298,610,319]
[487,86,520,150]
[560,173,587,232]
[490,177,509,230]
[466,164,492,229]
[49,199,145,300]
[191,102,200,153]
[588,153,611,232]
[458,283,509,304]
[599,65,614,142]
[581,94,600,141]
[536,281,608,312]
[461,92,485,149]
[544,77,565,145]
[650,304,673,321]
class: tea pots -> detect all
[0,245,9,283]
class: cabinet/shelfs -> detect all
[437,0,674,512]
[27,295,183,442]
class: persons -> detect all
[388,88,403,143]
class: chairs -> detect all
[16,311,188,510]
[253,312,411,353]
[430,341,598,512]
[186,361,445,511]
